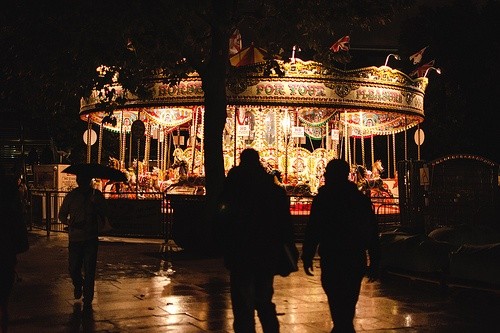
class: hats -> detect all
[325,160,349,170]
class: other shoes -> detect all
[74,279,84,299]
[84,297,92,308]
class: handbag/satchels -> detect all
[98,214,112,232]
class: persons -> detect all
[58,175,108,305]
[301,159,379,333]
[0,174,29,332]
[222,148,300,333]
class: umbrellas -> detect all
[61,163,128,181]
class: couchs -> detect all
[374,228,500,282]
